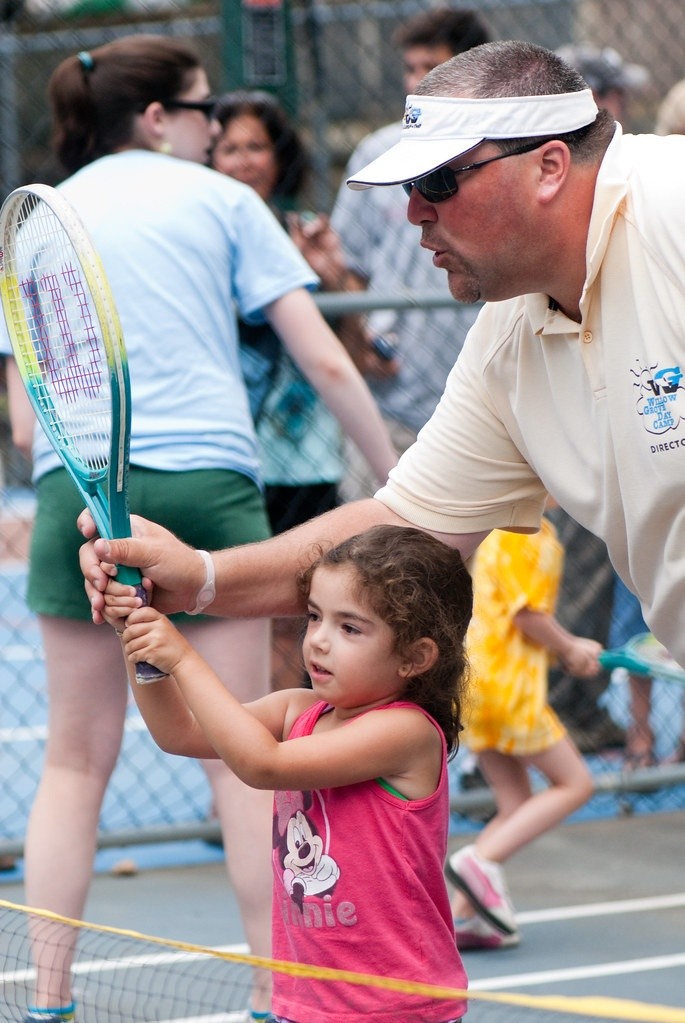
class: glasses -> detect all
[138,95,216,119]
[401,137,580,203]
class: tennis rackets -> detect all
[598,630,685,687]
[0,184,172,683]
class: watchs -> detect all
[184,550,217,616]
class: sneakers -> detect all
[451,918,522,949]
[447,845,516,935]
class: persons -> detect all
[443,511,604,951]
[98,520,476,1023]
[70,40,685,675]
[328,3,497,502]
[548,506,685,774]
[553,45,629,135]
[201,91,404,693]
[0,33,405,1023]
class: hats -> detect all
[346,87,597,193]
